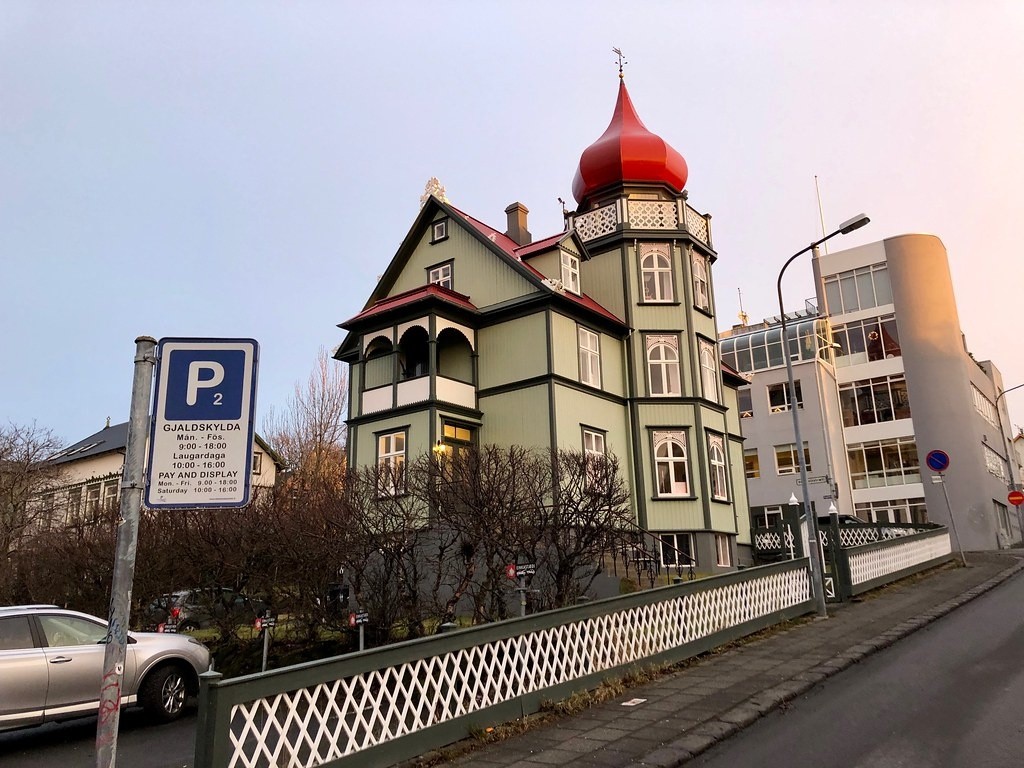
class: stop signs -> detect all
[1007,491,1024,506]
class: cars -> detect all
[818,512,870,527]
[1,601,215,742]
[143,588,266,633]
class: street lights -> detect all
[776,212,871,621]
